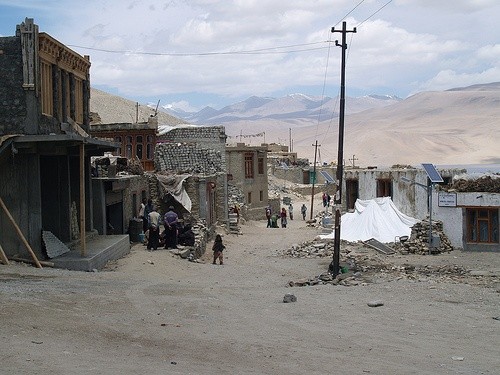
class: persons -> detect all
[272,213,280,228]
[301,204,307,220]
[138,197,195,250]
[280,207,287,229]
[266,207,272,228]
[212,234,226,265]
[322,192,331,207]
[289,204,293,220]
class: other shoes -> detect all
[220,262,223,264]
[213,261,216,264]
